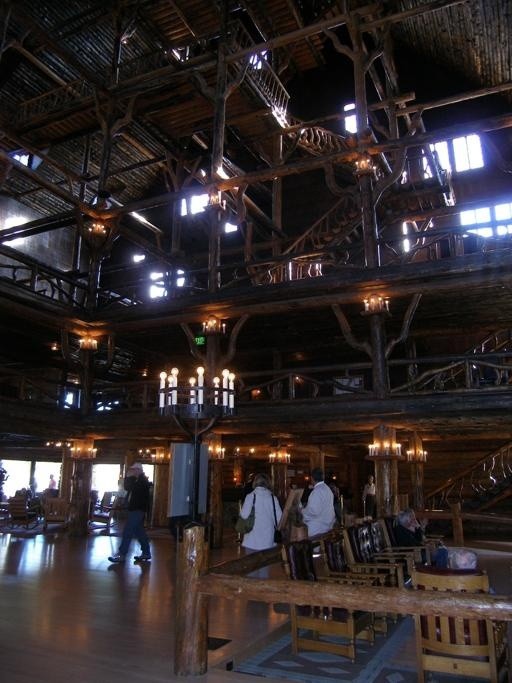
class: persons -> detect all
[395,508,449,570]
[329,480,341,522]
[361,475,376,519]
[296,468,337,536]
[301,475,315,509]
[240,474,282,579]
[109,463,153,562]
[283,480,303,542]
[240,473,255,503]
[48,474,57,490]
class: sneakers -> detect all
[134,551,151,561]
[108,551,125,562]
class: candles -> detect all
[69,446,100,460]
[269,453,291,463]
[188,377,197,404]
[197,367,204,404]
[222,369,229,406]
[368,443,402,456]
[213,377,220,405]
[228,373,235,408]
[159,372,167,407]
[355,158,376,171]
[202,319,226,334]
[363,297,389,312]
[145,448,172,465]
[167,375,173,405]
[89,223,106,234]
[208,446,226,459]
[407,450,427,462]
[78,339,98,349]
[171,367,179,405]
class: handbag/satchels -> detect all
[271,493,282,542]
[235,493,256,533]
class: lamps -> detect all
[159,366,236,676]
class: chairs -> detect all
[384,515,441,562]
[347,523,416,618]
[91,501,112,533]
[411,569,512,683]
[43,497,70,530]
[416,518,445,542]
[100,495,122,512]
[363,519,432,566]
[321,529,400,638]
[281,539,378,664]
[95,492,112,513]
[7,495,39,530]
[91,490,98,505]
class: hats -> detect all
[130,461,142,469]
